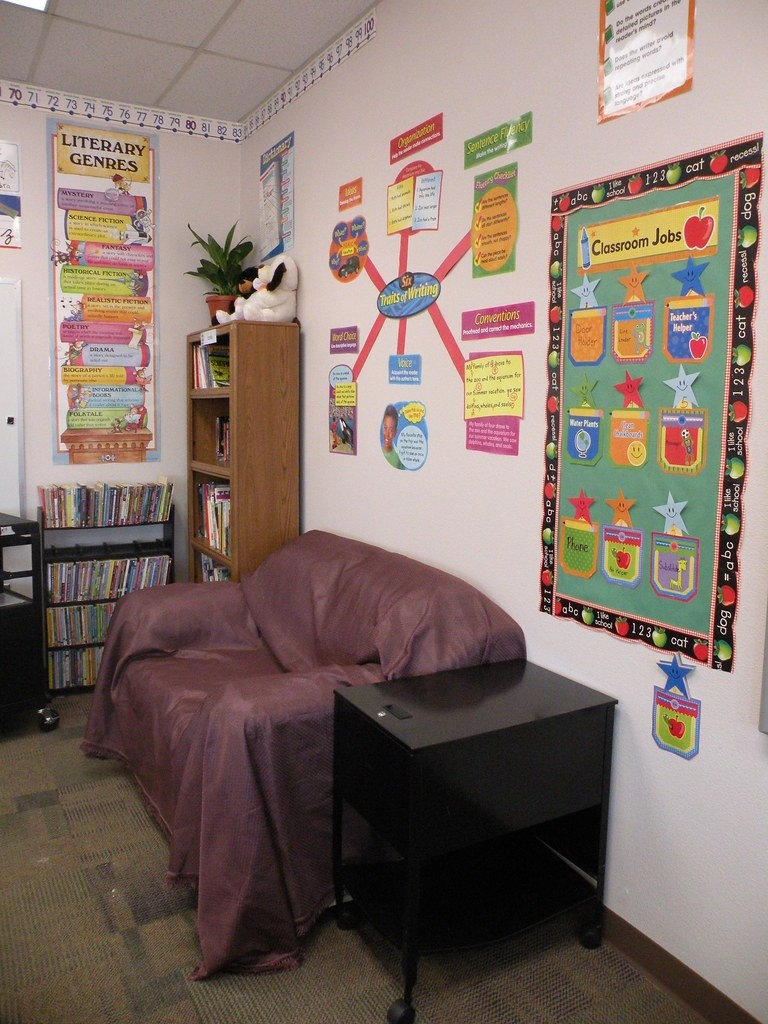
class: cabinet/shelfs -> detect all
[333,659,618,1024]
[0,512,61,733]
[187,319,301,583]
[36,503,175,697]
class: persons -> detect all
[382,405,403,469]
[330,418,352,448]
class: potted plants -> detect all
[183,218,253,327]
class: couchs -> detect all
[79,530,528,982]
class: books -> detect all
[192,347,229,583]
[37,474,175,688]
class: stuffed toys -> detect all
[215,253,299,324]
